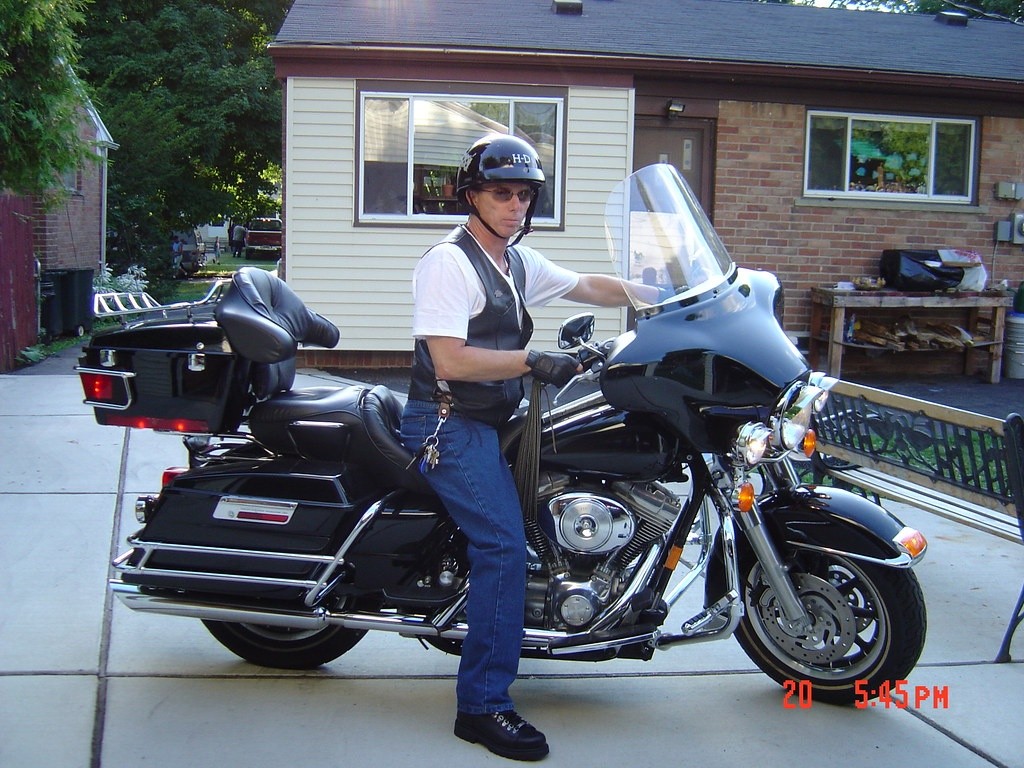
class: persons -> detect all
[171,235,188,281]
[230,217,248,258]
[397,132,702,762]
[214,236,221,265]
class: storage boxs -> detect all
[74,313,251,434]
[121,458,354,600]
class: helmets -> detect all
[456,135,546,204]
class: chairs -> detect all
[995,413,1024,664]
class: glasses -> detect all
[467,187,536,202]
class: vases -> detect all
[441,184,454,196]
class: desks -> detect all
[396,195,460,214]
[808,284,1015,383]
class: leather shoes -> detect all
[454,703,550,760]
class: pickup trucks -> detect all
[228,217,283,260]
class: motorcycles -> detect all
[74,163,928,709]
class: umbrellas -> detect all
[361,97,556,176]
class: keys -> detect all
[402,441,440,475]
